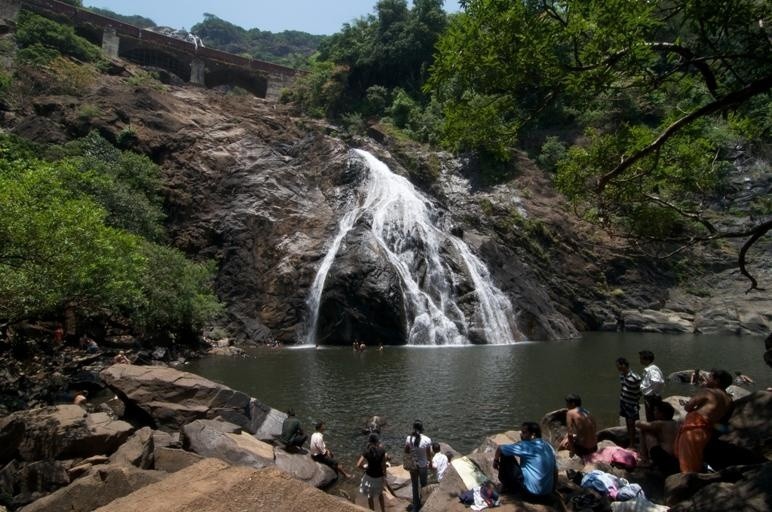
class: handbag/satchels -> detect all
[402,449,418,473]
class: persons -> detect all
[51,322,134,405]
[359,341,368,352]
[279,407,309,454]
[559,334,772,510]
[355,415,558,511]
[378,342,384,352]
[310,419,355,480]
[352,338,359,351]
